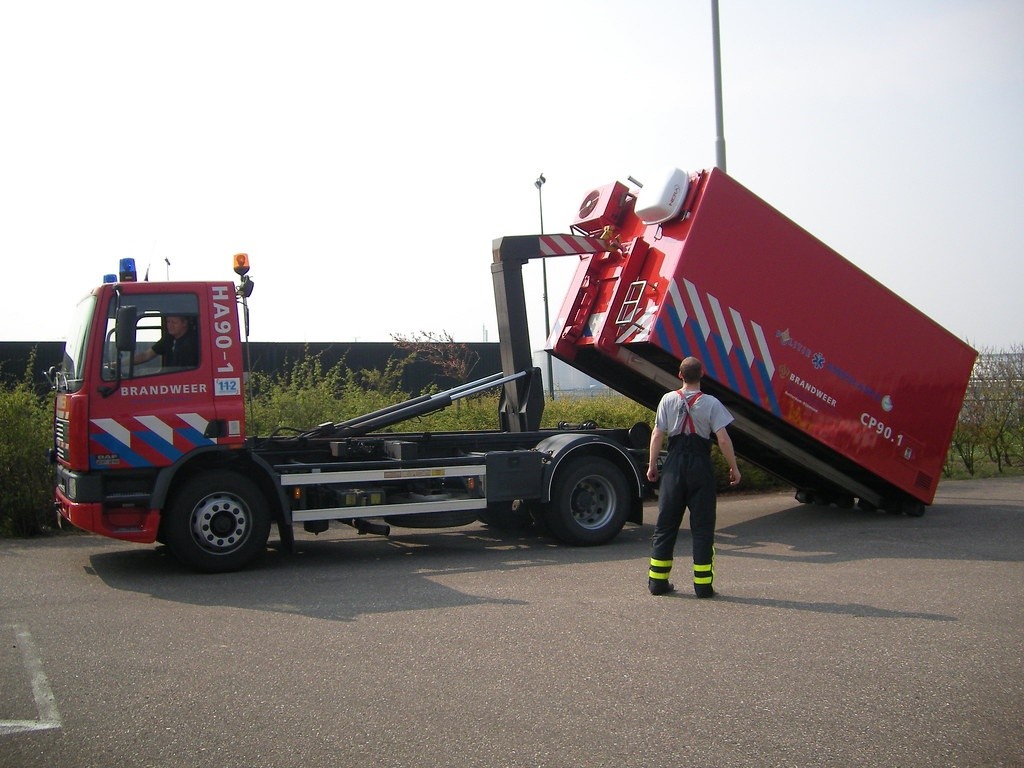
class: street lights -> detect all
[533,173,557,401]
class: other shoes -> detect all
[653,583,674,595]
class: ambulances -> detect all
[44,162,980,573]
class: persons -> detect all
[102,313,199,372]
[643,357,742,598]
